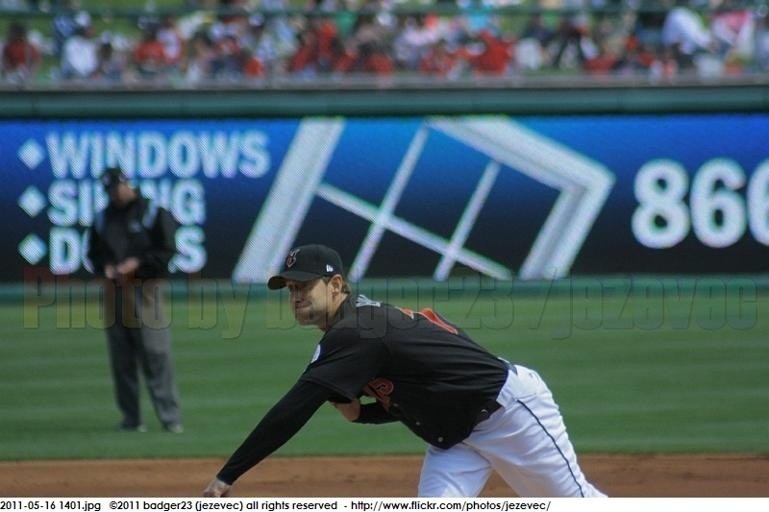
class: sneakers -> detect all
[123,423,146,433]
[163,423,184,433]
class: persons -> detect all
[1,1,769,92]
[85,165,182,433]
[203,243,610,498]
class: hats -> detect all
[267,243,344,290]
[99,169,129,191]
